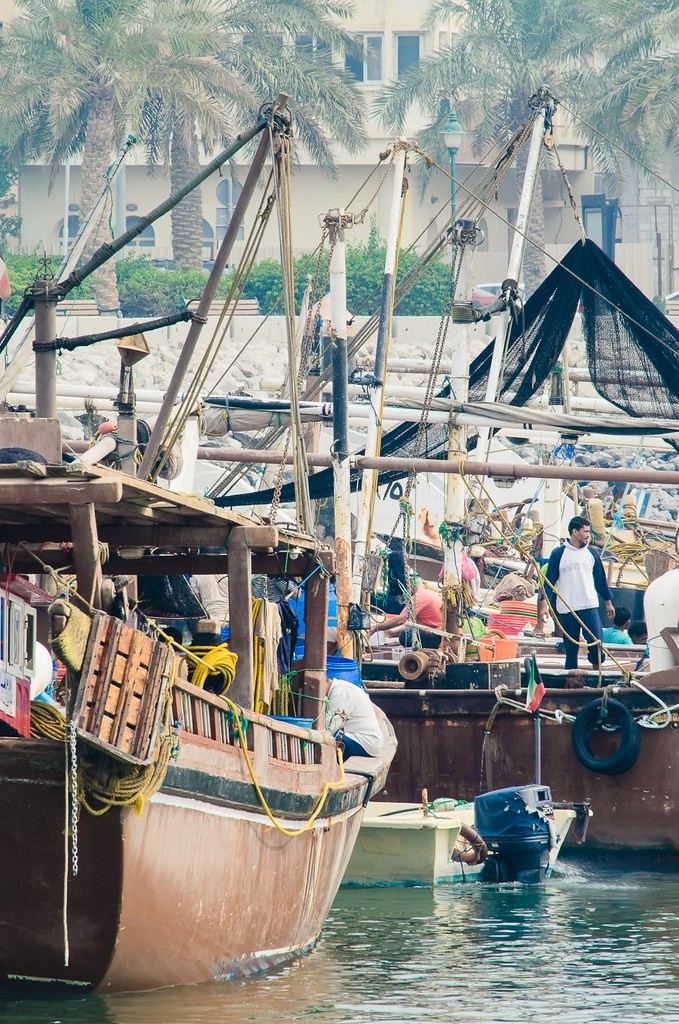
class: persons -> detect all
[325,677,382,761]
[366,577,443,648]
[190,574,230,640]
[462,607,486,654]
[602,607,634,643]
[540,516,615,669]
[268,577,301,603]
[628,622,649,644]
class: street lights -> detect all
[440,111,466,308]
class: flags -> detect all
[525,657,545,713]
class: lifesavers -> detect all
[439,817,488,867]
[571,696,642,778]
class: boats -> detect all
[0,84,401,999]
[183,80,679,869]
